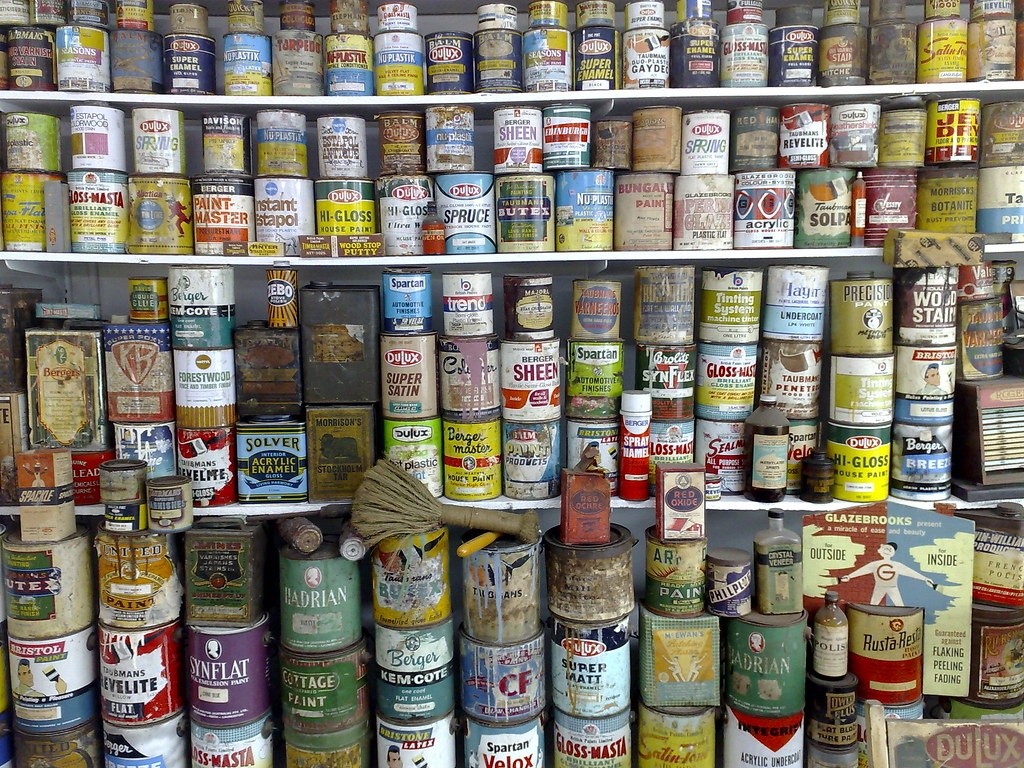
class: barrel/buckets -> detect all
[0,2,1024,768]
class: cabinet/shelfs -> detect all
[0,78,1024,519]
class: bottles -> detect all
[744,395,789,502]
[800,448,835,503]
[754,507,802,616]
[813,591,848,680]
